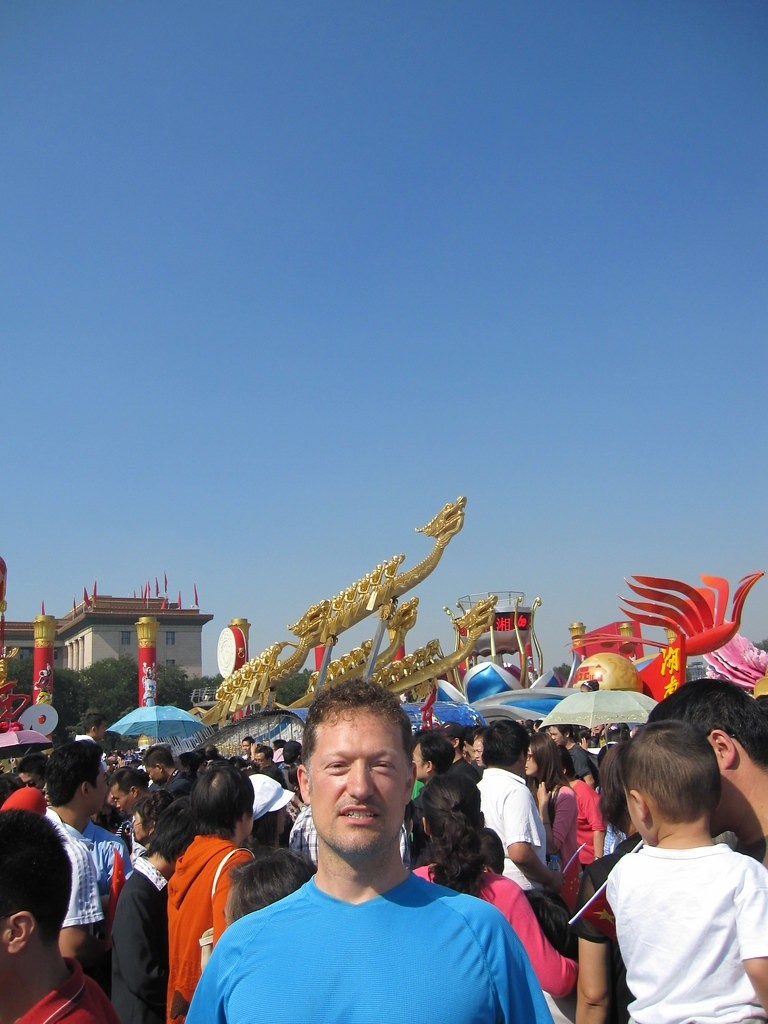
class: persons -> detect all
[184,678,556,1024]
[0,678,768,1024]
[604,720,768,1023]
[0,809,120,1024]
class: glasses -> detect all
[25,774,46,788]
[526,754,533,761]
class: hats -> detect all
[437,722,465,740]
[248,774,297,820]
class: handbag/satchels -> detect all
[199,848,255,975]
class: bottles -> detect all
[547,854,561,872]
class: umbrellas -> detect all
[0,730,53,760]
[538,689,658,730]
[105,706,208,743]
